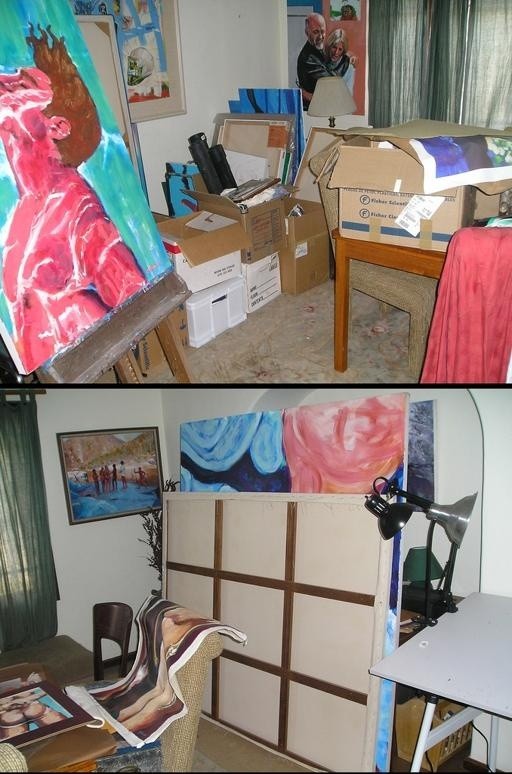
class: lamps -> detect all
[307,76,357,128]
[362,476,479,630]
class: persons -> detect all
[297,12,357,110]
[0,689,69,740]
[91,460,148,495]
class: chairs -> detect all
[158,631,224,772]
[92,601,133,681]
[308,137,438,376]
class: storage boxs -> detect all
[314,133,512,253]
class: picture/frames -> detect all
[75,14,140,178]
[55,425,165,525]
[0,680,96,750]
[211,113,297,186]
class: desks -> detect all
[330,226,447,372]
[366,592,512,773]
